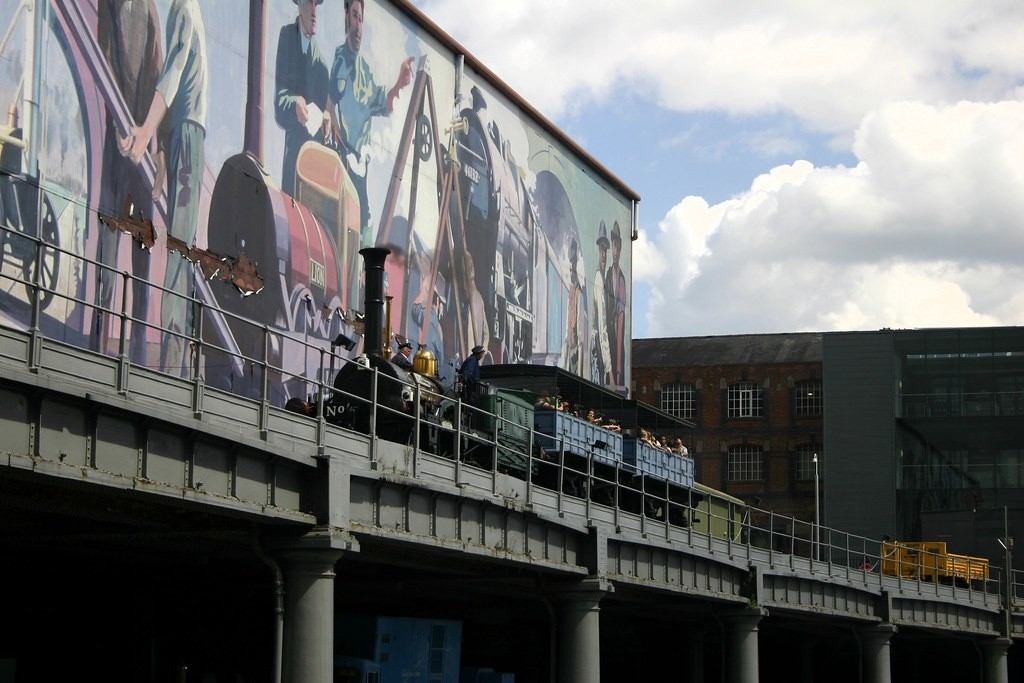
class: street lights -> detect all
[812,454,820,561]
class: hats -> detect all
[472,345,485,354]
[563,402,569,407]
[398,342,413,350]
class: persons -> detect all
[459,346,485,399]
[624,428,688,457]
[534,383,621,431]
[391,343,417,372]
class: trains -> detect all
[0,103,60,309]
[333,247,702,527]
[196,50,572,425]
